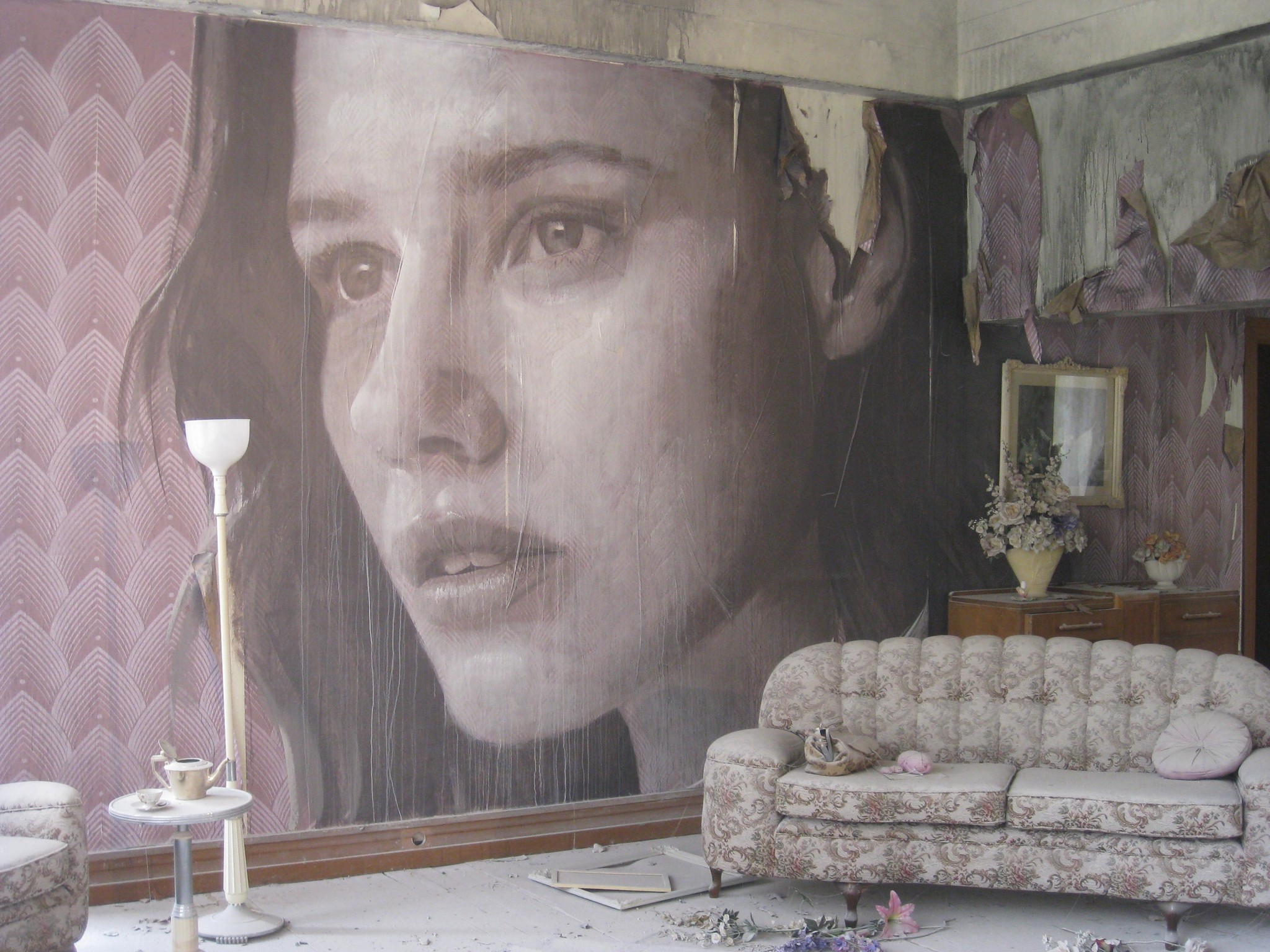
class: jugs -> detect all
[151,739,230,800]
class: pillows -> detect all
[1152,710,1251,779]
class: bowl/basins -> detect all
[136,789,163,805]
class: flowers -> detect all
[967,441,1087,558]
[1043,926,1211,952]
[659,890,920,952]
[1133,529,1190,564]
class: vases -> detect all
[1143,559,1187,589]
[1004,543,1066,599]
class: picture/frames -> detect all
[998,355,1126,509]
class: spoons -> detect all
[145,801,167,810]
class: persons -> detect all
[97,0,984,834]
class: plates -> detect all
[130,799,170,811]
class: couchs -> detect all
[0,781,88,952]
[700,634,1270,950]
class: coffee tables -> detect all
[109,786,254,952]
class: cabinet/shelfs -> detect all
[948,583,1241,656]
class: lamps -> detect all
[182,420,286,943]
